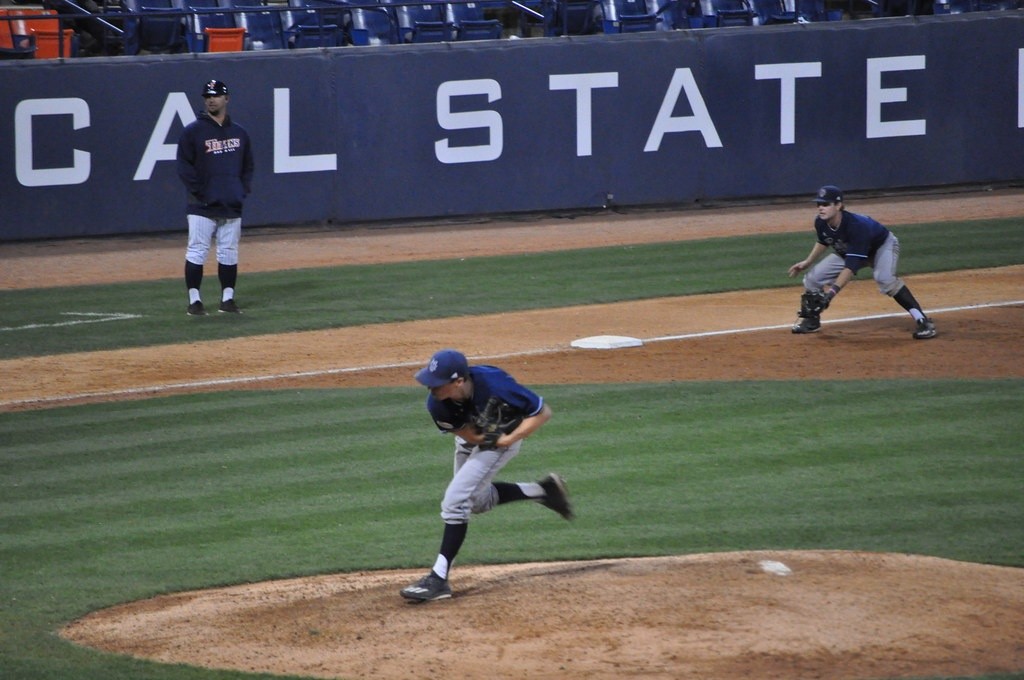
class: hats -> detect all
[812,185,843,203]
[201,81,228,99]
[413,351,467,388]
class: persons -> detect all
[399,350,574,602]
[788,185,936,340]
[177,80,254,316]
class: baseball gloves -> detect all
[798,290,831,318]
[476,398,521,434]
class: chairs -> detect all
[0,0,1024,58]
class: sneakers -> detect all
[219,300,243,315]
[791,322,821,335]
[536,473,572,521]
[399,572,451,600]
[187,302,209,317]
[913,320,937,339]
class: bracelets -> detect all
[830,283,840,295]
